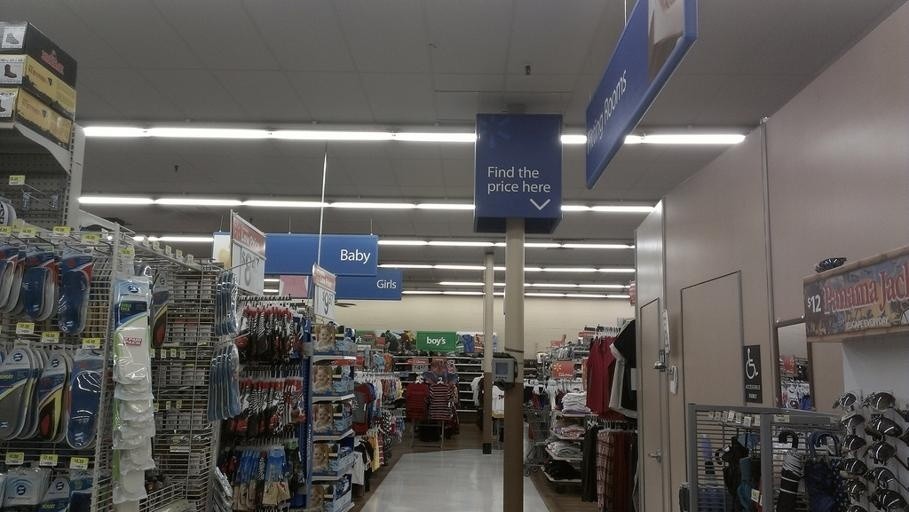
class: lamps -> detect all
[73,0,745,298]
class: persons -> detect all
[312,407,330,432]
[309,486,323,512]
[312,445,329,474]
[316,326,334,348]
[313,367,331,392]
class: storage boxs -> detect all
[0,19,79,152]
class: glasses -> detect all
[815,258,846,273]
[840,393,907,512]
